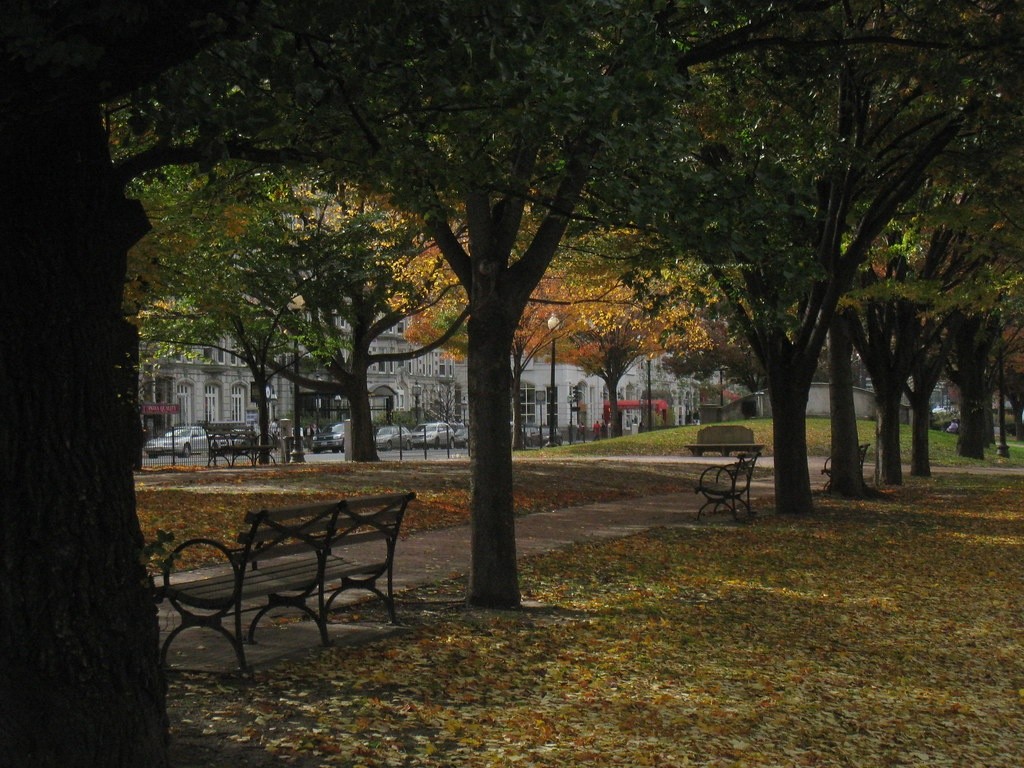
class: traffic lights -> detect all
[572,385,579,399]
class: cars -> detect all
[450,422,468,448]
[411,421,456,449]
[312,421,345,454]
[373,426,413,450]
[145,425,209,459]
[210,428,250,457]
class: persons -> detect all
[592,421,600,441]
[303,423,321,449]
[270,419,280,450]
[578,422,585,443]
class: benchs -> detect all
[820,443,871,493]
[201,426,279,469]
[683,424,766,456]
[154,490,416,676]
[692,452,762,523]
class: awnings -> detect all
[139,403,179,415]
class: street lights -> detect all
[314,375,322,433]
[412,380,423,426]
[991,306,1011,459]
[548,312,562,449]
[567,392,577,445]
[334,395,342,422]
